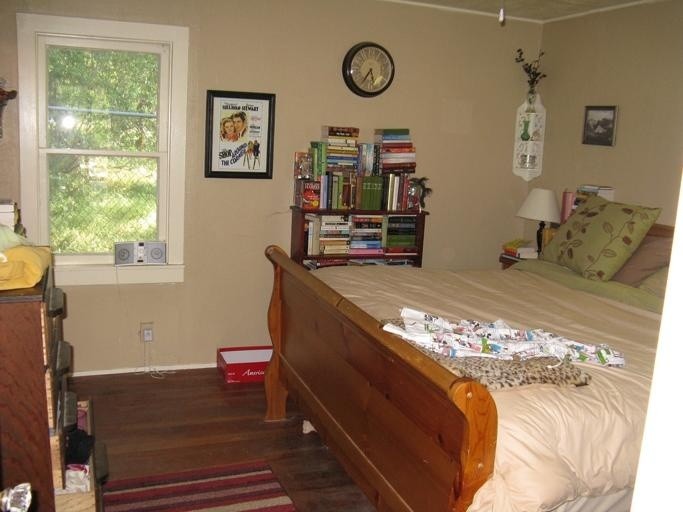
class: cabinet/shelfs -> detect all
[288,204,430,270]
[505,91,548,182]
[0,245,100,512]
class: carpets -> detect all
[102,454,297,512]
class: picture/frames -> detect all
[203,87,274,180]
[580,105,617,148]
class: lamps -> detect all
[514,187,561,254]
[494,7,508,23]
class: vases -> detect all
[523,85,538,112]
[520,120,530,141]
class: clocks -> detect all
[341,42,397,99]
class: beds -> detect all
[258,222,673,512]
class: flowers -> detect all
[511,45,548,93]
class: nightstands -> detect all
[494,251,524,269]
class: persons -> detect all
[218,112,261,170]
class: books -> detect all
[294,126,421,270]
[502,184,614,260]
[0,200,20,238]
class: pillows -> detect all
[538,194,662,280]
[611,236,672,285]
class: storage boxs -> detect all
[215,344,273,385]
[0,198,16,234]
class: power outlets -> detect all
[139,319,154,345]
